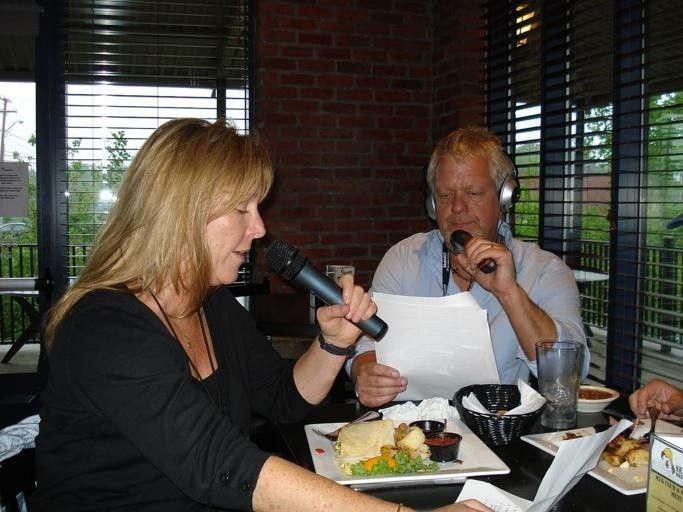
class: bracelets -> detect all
[316,332,355,357]
[397,503,402,512]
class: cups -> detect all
[421,431,461,462]
[410,419,443,438]
[533,341,584,429]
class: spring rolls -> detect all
[337,419,395,459]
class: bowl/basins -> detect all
[577,385,619,413]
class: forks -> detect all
[311,410,380,441]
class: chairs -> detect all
[0,401,45,509]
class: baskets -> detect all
[453,383,545,445]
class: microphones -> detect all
[263,239,389,341]
[449,229,495,273]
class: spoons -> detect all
[643,407,661,444]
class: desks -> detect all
[567,269,609,346]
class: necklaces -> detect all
[173,316,199,347]
[143,279,222,415]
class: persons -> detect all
[341,125,592,407]
[26,116,492,512]
[628,378,682,422]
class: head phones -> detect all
[424,149,521,219]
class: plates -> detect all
[521,416,682,497]
[301,419,511,492]
